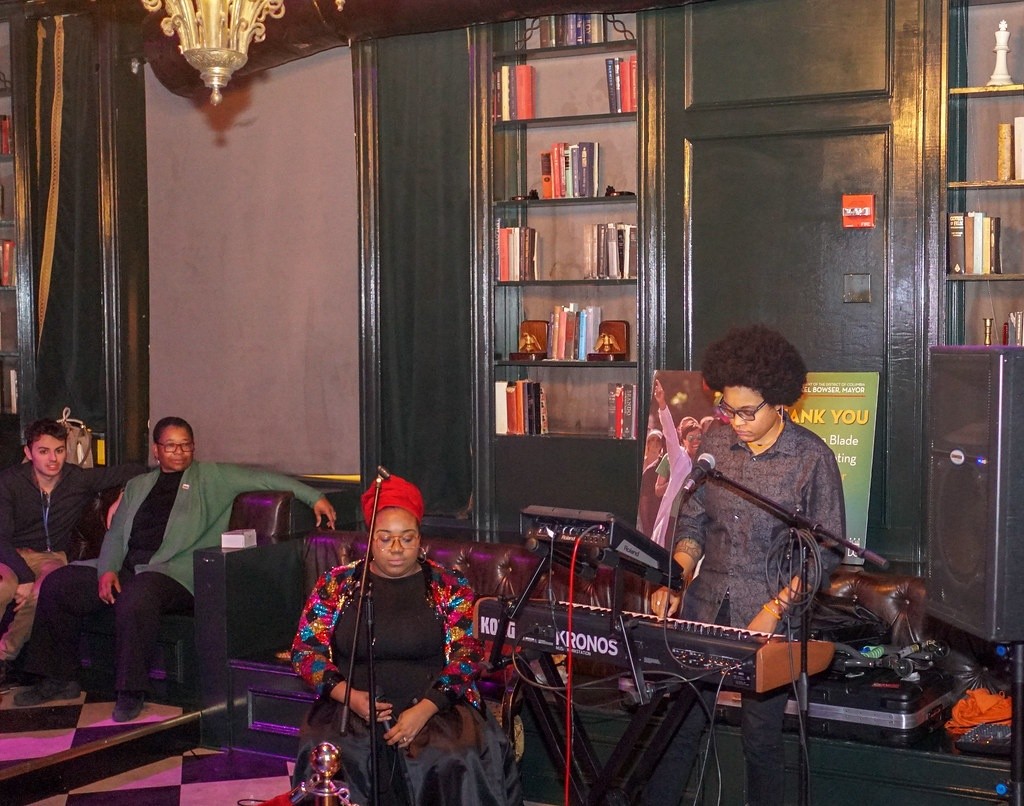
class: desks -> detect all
[522,680,1011,806]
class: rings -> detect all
[656,601,661,606]
[403,737,406,742]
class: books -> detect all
[499,226,541,281]
[605,55,638,112]
[492,64,537,121]
[541,141,600,199]
[539,13,608,49]
[545,302,603,362]
[607,382,639,440]
[582,221,637,279]
[997,116,1024,182]
[494,378,549,436]
[948,212,1004,275]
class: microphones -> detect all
[376,685,398,752]
[684,453,716,493]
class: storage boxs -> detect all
[221,530,257,550]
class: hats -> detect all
[360,474,424,528]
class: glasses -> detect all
[373,534,419,550]
[157,443,194,453]
[717,397,770,421]
[686,434,702,442]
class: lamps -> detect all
[141,0,348,106]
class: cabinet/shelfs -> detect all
[492,36,638,369]
[940,1,1024,347]
[0,16,27,413]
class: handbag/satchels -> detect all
[59,420,93,468]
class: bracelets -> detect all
[763,597,787,620]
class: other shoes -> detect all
[112,690,145,721]
[14,678,81,706]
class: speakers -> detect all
[924,343,1024,646]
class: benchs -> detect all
[191,530,934,755]
[0,466,359,703]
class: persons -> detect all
[627,321,847,806]
[13,416,338,723]
[638,379,713,552]
[288,473,525,806]
[0,415,157,693]
